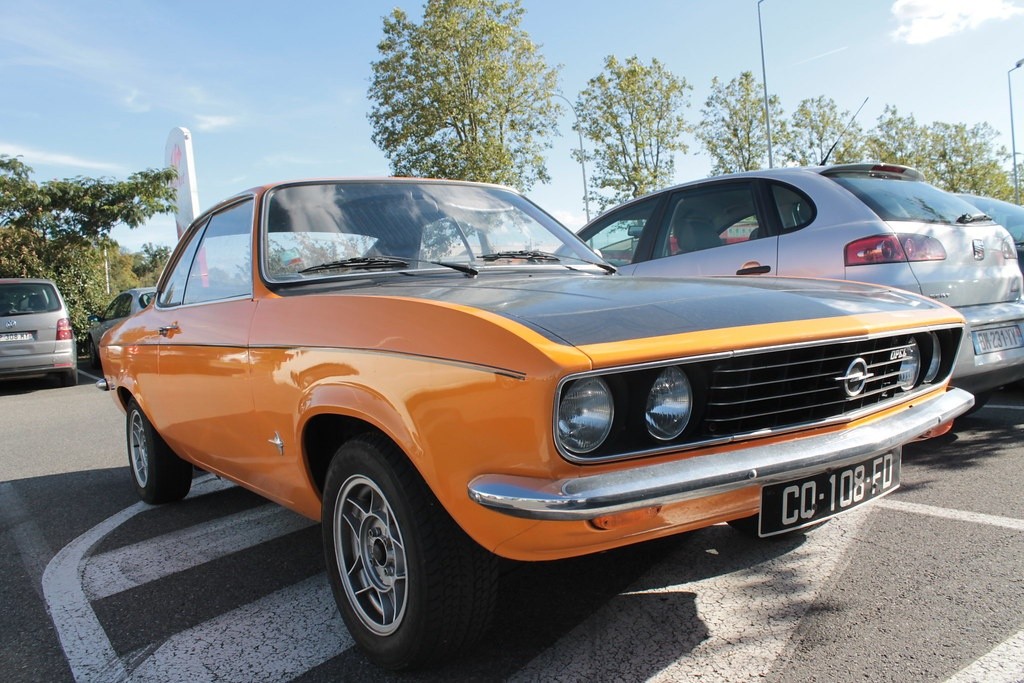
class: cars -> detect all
[0,277,79,386]
[951,191,1024,275]
[548,159,1024,417]
[87,285,156,371]
[96,177,977,669]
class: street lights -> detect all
[1007,58,1024,206]
[540,92,594,250]
